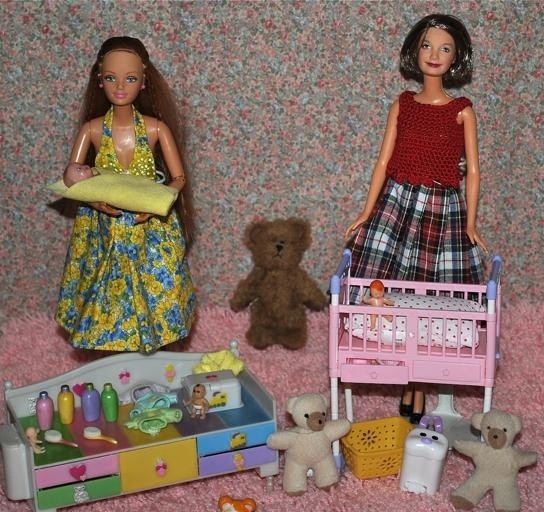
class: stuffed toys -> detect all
[264,389,351,494]
[227,214,323,350]
[448,408,538,512]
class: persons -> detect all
[340,13,492,426]
[61,162,100,187]
[52,35,200,355]
